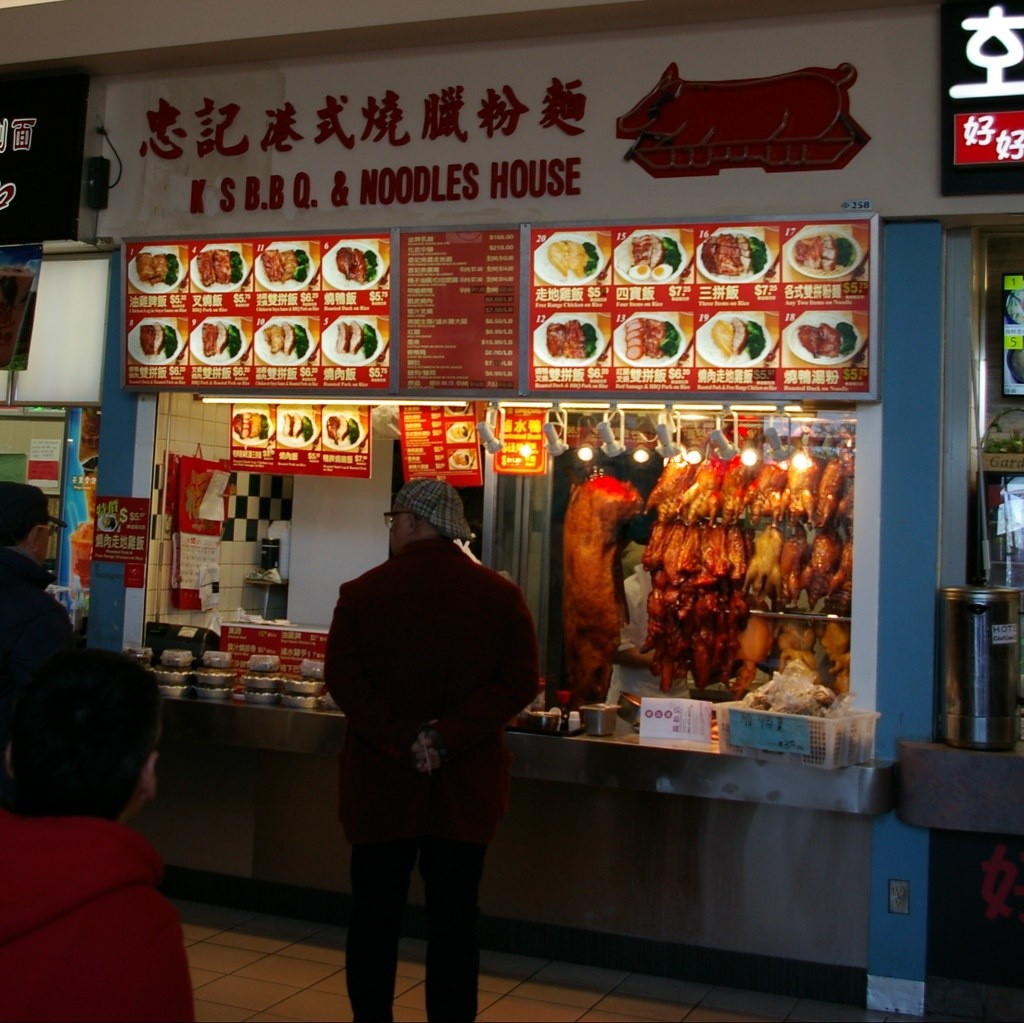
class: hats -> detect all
[0,480,68,538]
[395,478,472,540]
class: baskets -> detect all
[710,700,881,770]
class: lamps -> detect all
[577,415,602,462]
[765,406,795,462]
[476,403,506,453]
[709,405,740,460]
[654,404,681,456]
[541,402,569,455]
[597,404,626,457]
[629,415,652,465]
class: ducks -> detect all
[641,450,854,700]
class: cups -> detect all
[261,538,279,571]
[80,465,97,523]
[70,535,93,591]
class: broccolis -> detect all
[260,414,359,444]
[582,234,857,359]
[164,250,379,359]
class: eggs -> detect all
[629,264,651,280]
[652,264,674,281]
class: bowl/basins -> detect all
[125,648,325,709]
[616,690,641,726]
[528,712,559,732]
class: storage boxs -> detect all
[712,701,881,771]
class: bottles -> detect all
[568,711,580,734]
[533,677,547,712]
[557,691,574,732]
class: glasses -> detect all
[36,524,56,536]
[384,511,414,529]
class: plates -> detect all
[533,310,861,369]
[445,405,475,469]
[129,241,385,294]
[128,316,384,367]
[534,231,861,284]
[232,408,366,450]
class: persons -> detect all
[617,521,656,577]
[604,560,693,733]
[0,647,195,1022]
[0,480,73,643]
[324,480,538,1023]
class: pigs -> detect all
[562,475,642,704]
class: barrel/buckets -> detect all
[941,574,1023,753]
